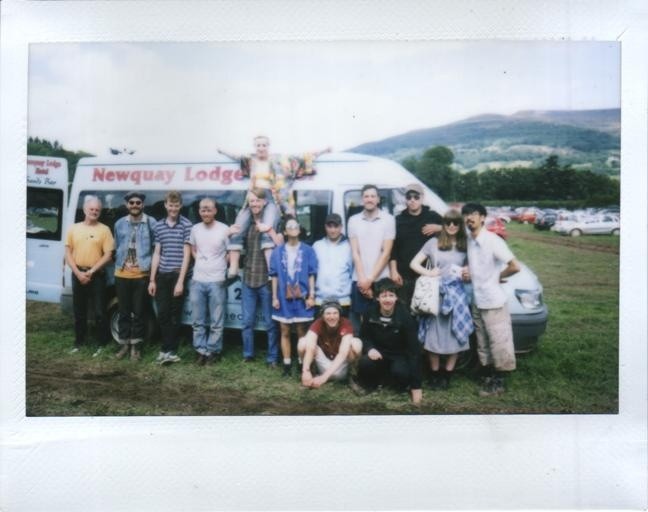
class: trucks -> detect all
[26,147,551,364]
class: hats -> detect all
[405,182,424,195]
[124,191,147,201]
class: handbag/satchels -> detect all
[409,237,439,317]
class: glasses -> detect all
[406,195,420,201]
[129,200,142,205]
[447,221,459,226]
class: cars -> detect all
[35,206,59,216]
[449,204,620,240]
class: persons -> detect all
[63,194,116,359]
[267,217,321,379]
[225,185,285,370]
[346,183,397,321]
[295,300,365,396]
[112,190,157,363]
[357,277,424,404]
[145,188,194,366]
[215,133,334,290]
[461,201,521,398]
[308,211,354,319]
[407,207,475,391]
[389,183,444,308]
[188,196,232,367]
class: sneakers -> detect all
[426,368,457,392]
[68,339,223,367]
[481,375,509,398]
[280,362,293,380]
[237,355,279,371]
